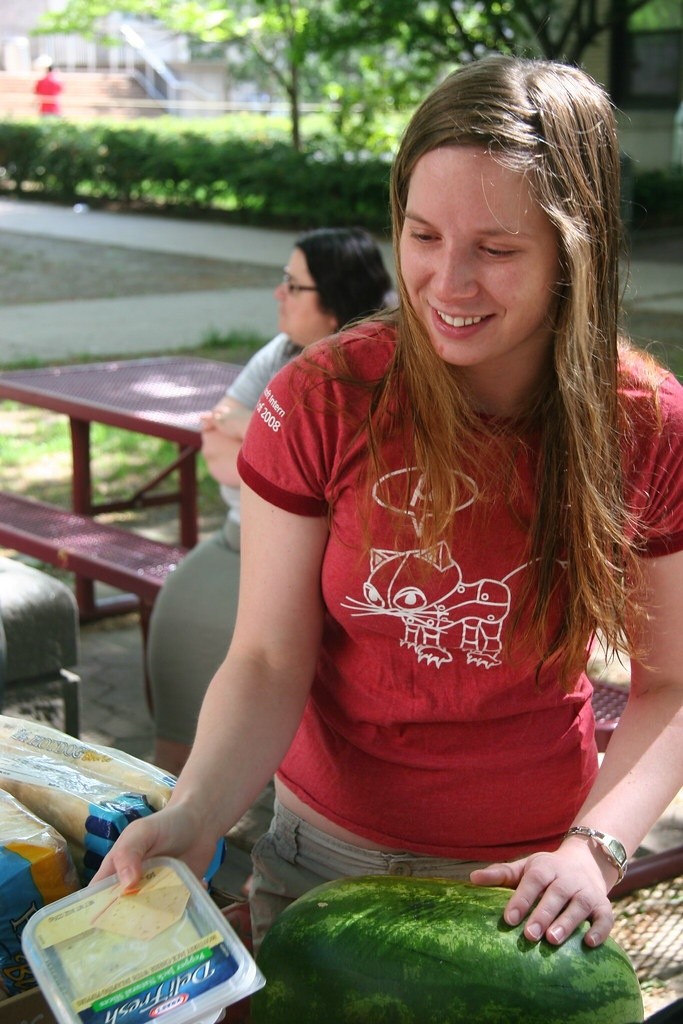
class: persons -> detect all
[80,54,683,953]
[151,226,391,847]
[35,67,62,114]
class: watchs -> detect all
[563,823,629,885]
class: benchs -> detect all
[0,492,193,718]
[590,680,633,752]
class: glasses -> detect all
[280,273,318,295]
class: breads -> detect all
[0,719,184,995]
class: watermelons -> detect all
[246,874,648,1024]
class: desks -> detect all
[0,355,247,623]
